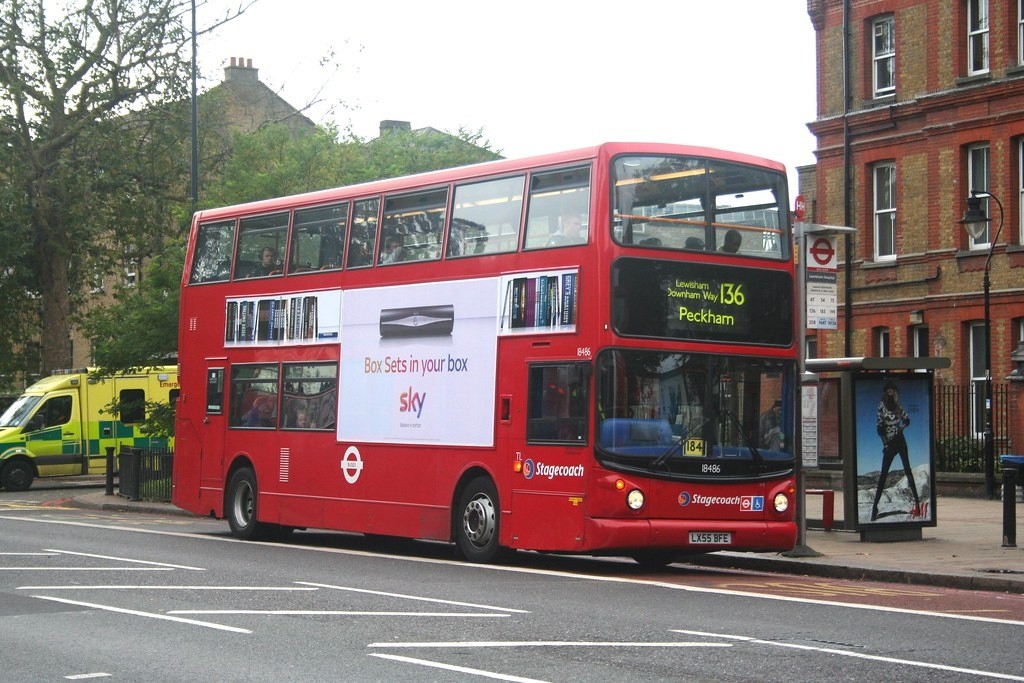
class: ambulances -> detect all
[0,364,179,492]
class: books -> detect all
[227,296,339,341]
[499,270,579,329]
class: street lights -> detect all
[956,188,1004,500]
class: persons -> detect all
[253,246,282,276]
[289,406,315,429]
[685,237,705,250]
[716,230,742,255]
[381,237,422,264]
[868,382,920,521]
[760,400,785,452]
[551,210,583,245]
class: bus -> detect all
[170,142,798,571]
